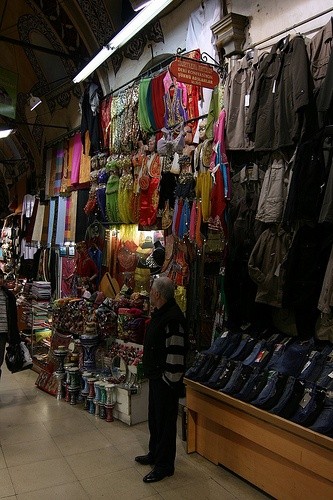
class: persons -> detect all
[135,276,186,483]
[0,269,21,377]
[152,241,166,266]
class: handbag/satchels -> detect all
[20,341,34,370]
[5,342,24,371]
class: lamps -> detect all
[29,93,42,111]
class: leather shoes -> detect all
[135,455,155,466]
[143,465,174,482]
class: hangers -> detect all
[245,24,303,61]
